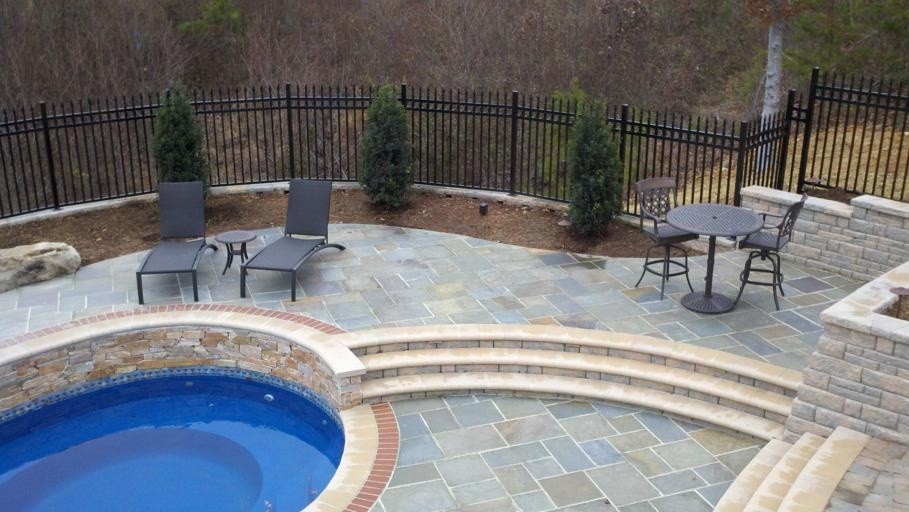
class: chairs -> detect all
[635,177,807,314]
[136,179,345,304]
